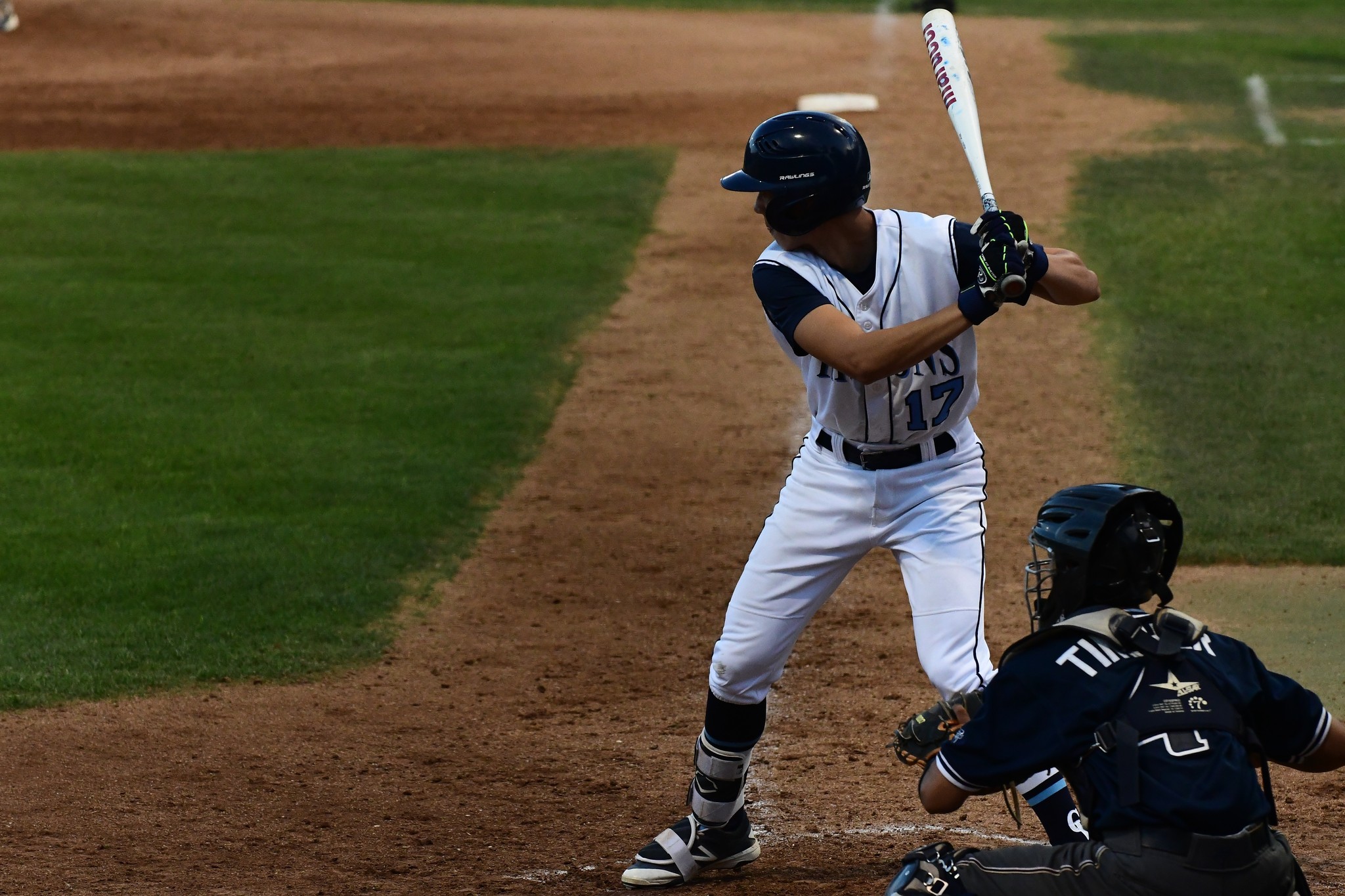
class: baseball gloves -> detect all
[892,689,985,770]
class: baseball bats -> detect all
[922,9,1027,299]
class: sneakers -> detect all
[621,809,760,888]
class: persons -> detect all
[887,481,1345,896]
[617,113,1102,889]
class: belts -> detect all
[1109,826,1277,868]
[815,423,956,471]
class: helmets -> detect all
[719,110,872,235]
[1024,482,1182,634]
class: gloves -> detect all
[957,234,1027,325]
[969,208,1050,287]
